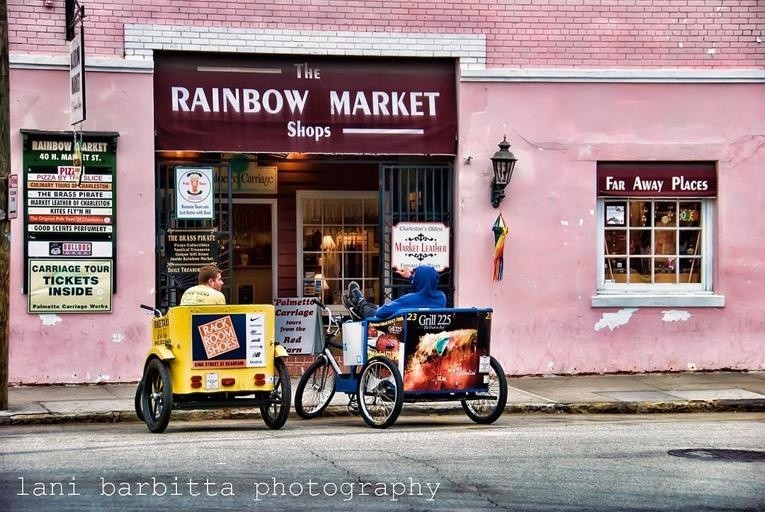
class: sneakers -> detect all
[342,295,361,321]
[349,281,366,306]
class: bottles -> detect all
[72,142,83,177]
[605,201,704,275]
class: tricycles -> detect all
[133,303,291,434]
[294,300,508,429]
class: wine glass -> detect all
[431,326,477,380]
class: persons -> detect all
[179,265,226,305]
[342,264,448,324]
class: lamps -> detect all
[320,235,336,263]
[490,133,518,209]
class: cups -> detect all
[190,175,200,192]
[240,253,249,267]
